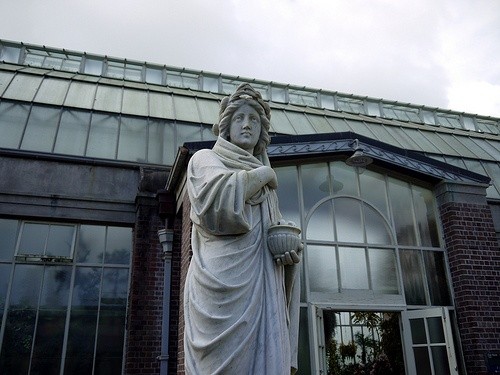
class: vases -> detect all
[267,223,302,260]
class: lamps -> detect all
[346,138,373,166]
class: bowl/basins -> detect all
[268,225,301,258]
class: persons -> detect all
[185,81,305,375]
